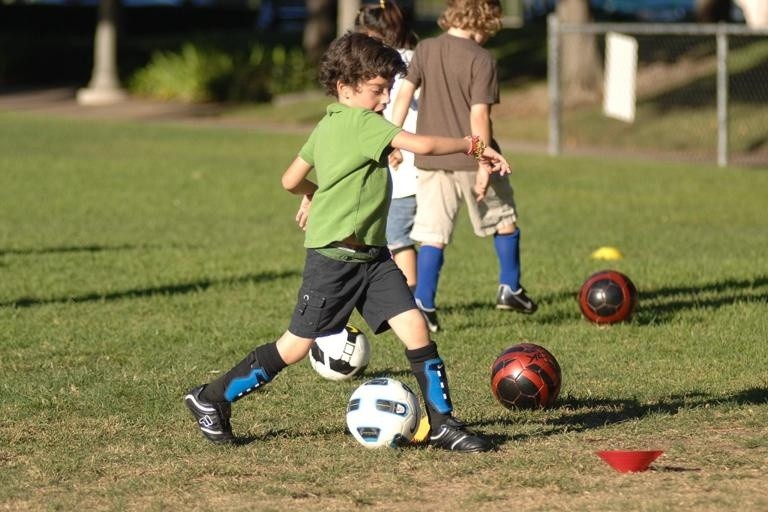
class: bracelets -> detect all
[464,135,484,159]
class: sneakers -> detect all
[415,298,442,334]
[184,384,240,446]
[426,416,486,452]
[495,284,539,315]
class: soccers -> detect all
[346,378,421,449]
[309,323,371,381]
[579,271,641,327]
[492,342,562,409]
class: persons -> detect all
[355,0,421,291]
[186,33,512,454]
[388,0,538,332]
